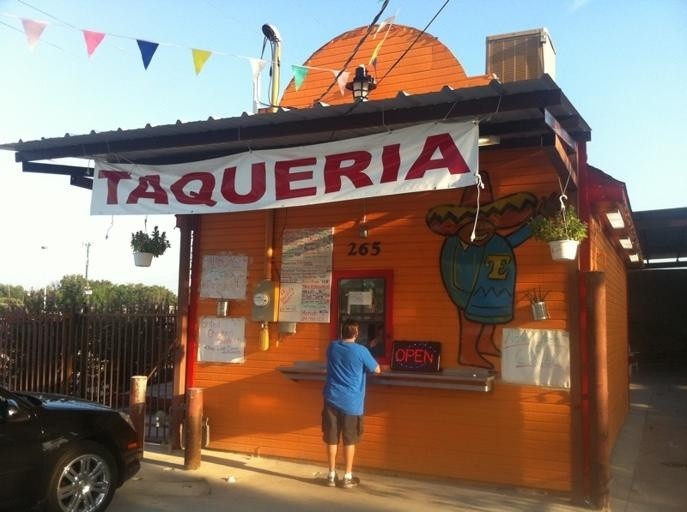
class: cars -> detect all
[0,379,146,512]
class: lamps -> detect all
[344,65,377,103]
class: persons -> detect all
[322,319,381,491]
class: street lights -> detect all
[41,246,48,315]
[81,241,92,278]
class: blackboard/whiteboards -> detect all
[197,316,246,365]
[391,341,441,372]
[199,254,249,300]
[278,226,335,324]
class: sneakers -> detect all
[327,473,360,488]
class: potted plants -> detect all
[528,204,587,263]
[129,227,169,267]
